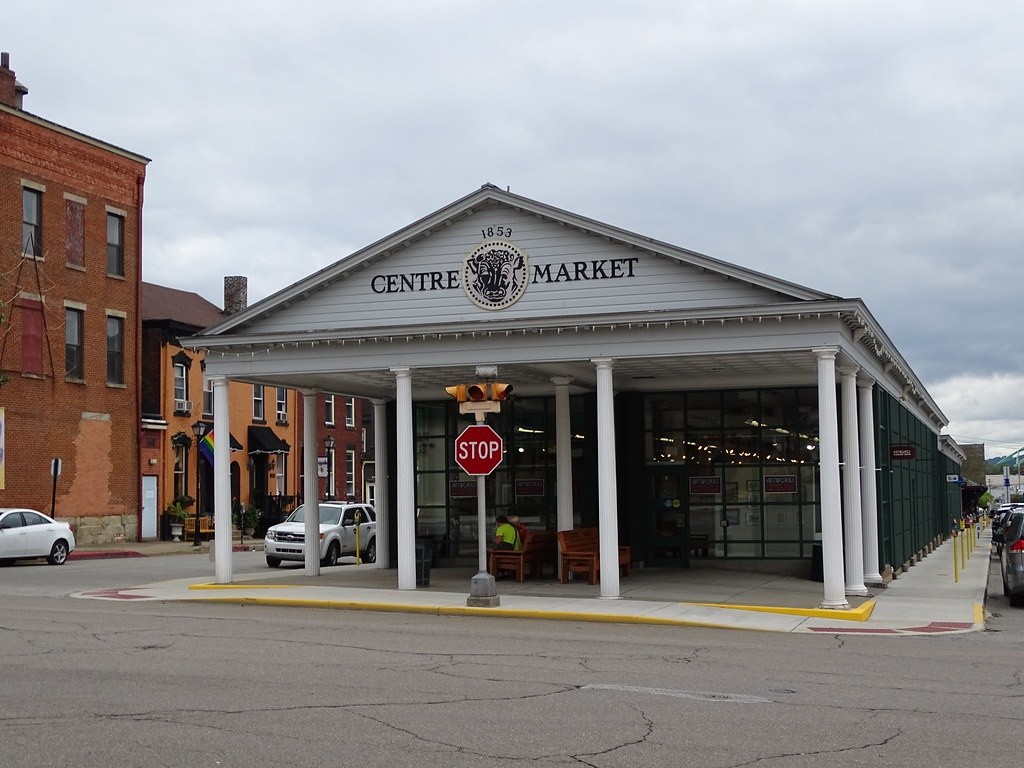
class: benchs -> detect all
[556,526,633,586]
[488,532,556,583]
[184,517,215,542]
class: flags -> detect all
[199,431,215,464]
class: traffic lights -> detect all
[491,382,513,401]
[445,383,467,403]
[466,383,488,402]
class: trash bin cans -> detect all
[811,541,823,582]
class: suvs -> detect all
[263,501,377,569]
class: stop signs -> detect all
[455,425,502,476]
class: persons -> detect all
[492,515,528,577]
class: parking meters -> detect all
[959,516,966,570]
[952,519,959,583]
[966,513,976,560]
[354,510,362,567]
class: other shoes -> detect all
[497,575,512,580]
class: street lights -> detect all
[324,433,336,501]
[191,420,206,547]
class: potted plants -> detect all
[233,503,264,536]
[165,502,188,542]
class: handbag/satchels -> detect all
[493,541,515,552]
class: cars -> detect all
[988,502,1024,607]
[0,507,75,565]
